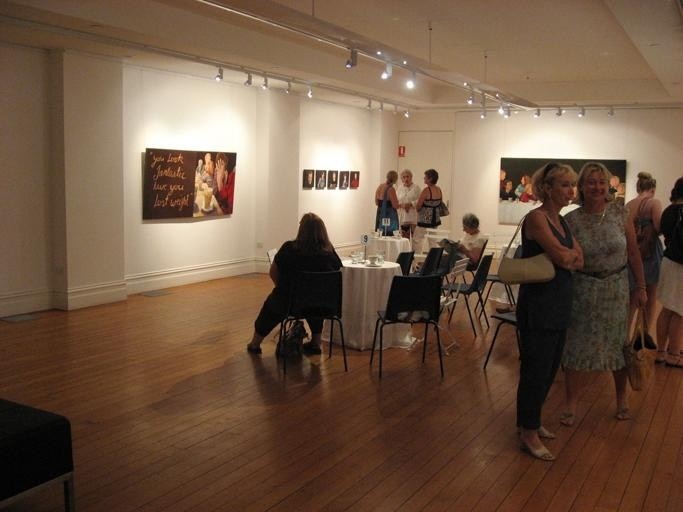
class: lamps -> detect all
[214,64,409,119]
[464,82,505,115]
[477,106,614,117]
[343,48,417,90]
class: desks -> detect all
[320,258,416,350]
[366,235,411,261]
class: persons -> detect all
[455,213,486,267]
[501,169,507,180]
[416,169,442,229]
[501,180,514,200]
[193,152,236,215]
[395,169,421,238]
[374,171,400,236]
[515,162,683,462]
[247,214,343,353]
[305,171,358,189]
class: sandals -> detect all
[656,351,683,368]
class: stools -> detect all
[0,399,76,512]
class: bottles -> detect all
[515,198,520,202]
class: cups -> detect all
[508,197,513,202]
[369,228,383,238]
[369,256,377,264]
[392,230,400,237]
[350,251,364,264]
[193,175,214,212]
[376,251,386,261]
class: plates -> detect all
[365,263,380,267]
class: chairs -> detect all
[369,274,445,380]
[437,254,453,312]
[413,227,450,271]
[444,239,488,285]
[396,251,415,277]
[438,257,470,357]
[483,312,523,368]
[277,271,348,376]
[434,252,495,337]
[419,247,443,273]
[473,245,523,320]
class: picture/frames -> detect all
[303,169,359,189]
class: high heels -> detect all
[518,431,558,462]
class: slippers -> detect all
[302,341,322,356]
[559,403,577,427]
[612,393,632,421]
[246,341,262,355]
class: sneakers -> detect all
[515,422,558,440]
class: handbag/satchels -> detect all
[438,200,449,217]
[496,250,559,287]
[276,321,307,362]
[623,328,659,392]
